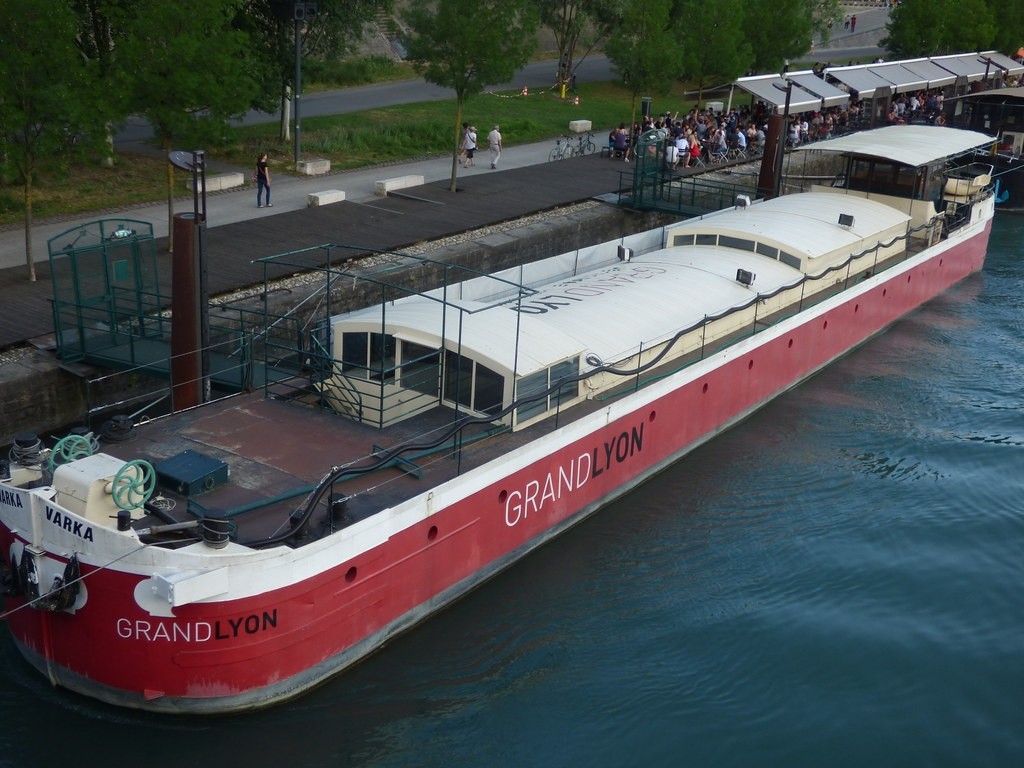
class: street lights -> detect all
[292,1,331,161]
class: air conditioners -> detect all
[1001,131,1024,154]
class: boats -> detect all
[936,85,1024,202]
[0,108,1001,711]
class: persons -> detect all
[844,15,850,31]
[827,19,832,31]
[256,153,274,208]
[610,58,1024,171]
[462,126,477,168]
[489,125,502,169]
[851,15,856,33]
[458,123,471,164]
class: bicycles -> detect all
[572,131,596,157]
[548,133,574,162]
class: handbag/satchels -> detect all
[476,146,478,150]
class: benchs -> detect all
[185,172,245,194]
[568,120,593,134]
[296,158,331,177]
[308,190,345,207]
[705,101,724,112]
[375,174,424,197]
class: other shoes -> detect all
[458,158,462,164]
[625,157,629,163]
[464,163,468,168]
[610,155,614,160]
[471,163,475,166]
[490,163,496,169]
[258,203,273,208]
[685,164,691,168]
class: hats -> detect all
[468,126,477,131]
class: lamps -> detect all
[733,194,752,209]
[838,213,857,232]
[735,269,756,289]
[617,245,633,263]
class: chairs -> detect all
[600,135,789,171]
